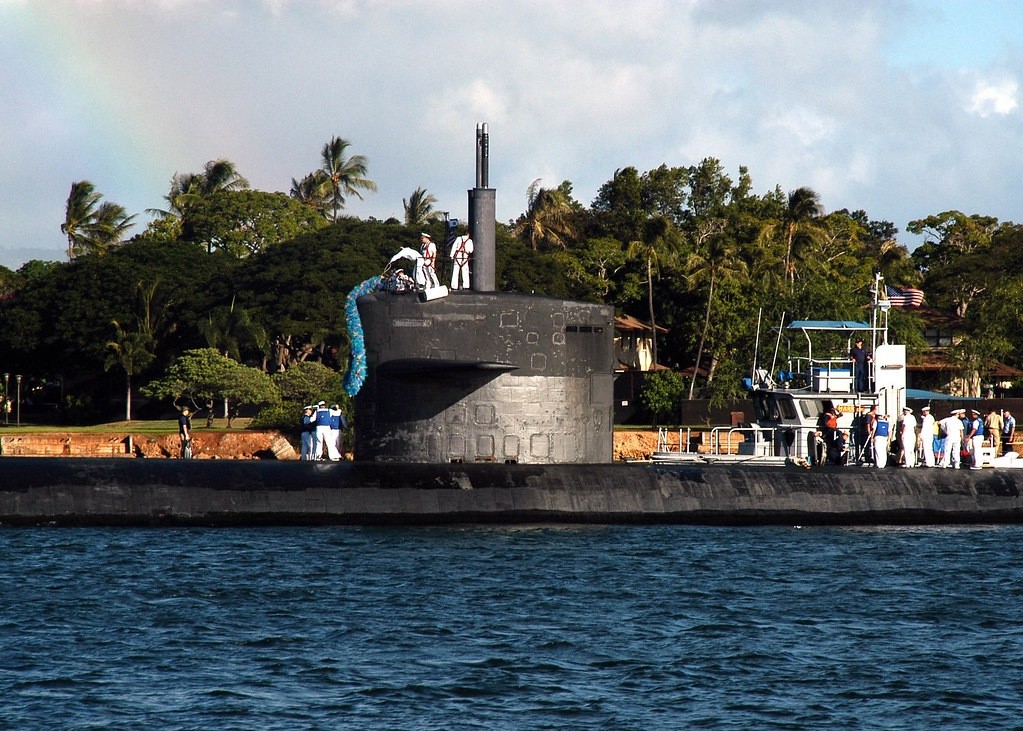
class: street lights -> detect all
[4,373,11,424]
[15,375,23,428]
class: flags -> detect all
[884,285,924,307]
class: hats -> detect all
[318,401,326,406]
[395,268,404,274]
[1002,408,1010,412]
[875,413,882,416]
[181,406,189,411]
[903,406,913,413]
[303,405,313,411]
[830,407,836,410]
[921,407,930,411]
[959,409,967,414]
[420,232,431,239]
[950,409,961,416]
[870,405,877,410]
[855,337,861,342]
[972,409,980,416]
[841,429,849,437]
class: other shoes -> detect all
[970,466,982,470]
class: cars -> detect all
[31,381,66,407]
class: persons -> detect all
[850,337,870,392]
[179,406,192,458]
[815,399,1015,469]
[301,401,347,461]
[388,233,439,294]
[450,229,474,290]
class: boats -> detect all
[650,271,995,464]
